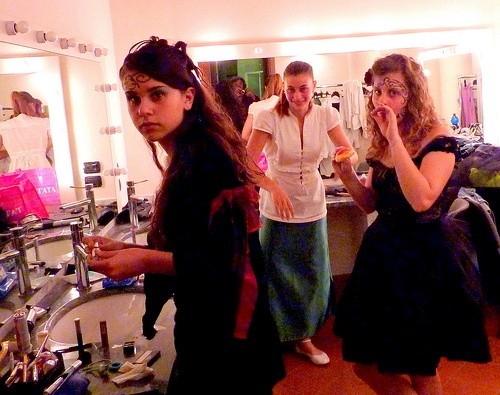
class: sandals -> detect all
[294,339,330,365]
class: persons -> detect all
[246,61,359,365]
[80,36,287,395]
[334,54,491,395]
[240,73,286,172]
[0,90,55,174]
[229,75,259,109]
[32,98,45,119]
[215,80,246,138]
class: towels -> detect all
[318,80,368,178]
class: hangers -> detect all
[311,85,372,103]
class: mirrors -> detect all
[0,41,119,223]
[195,43,483,177]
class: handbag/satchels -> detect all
[2,172,49,224]
